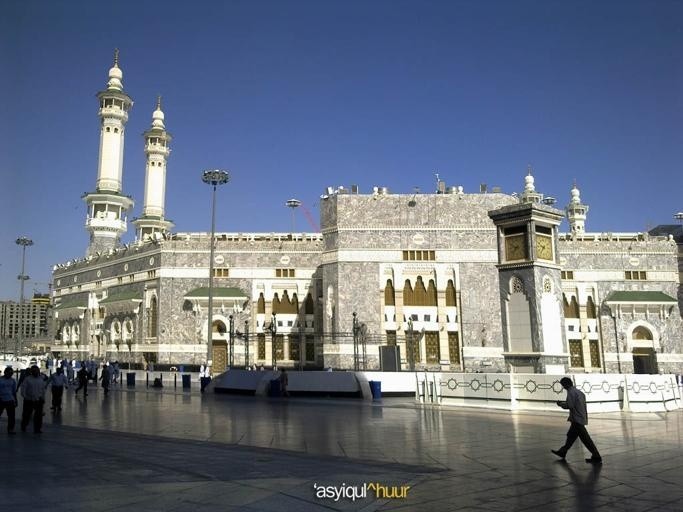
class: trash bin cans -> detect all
[182,374,191,388]
[127,373,135,387]
[200,377,211,394]
[368,381,381,400]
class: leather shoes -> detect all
[551,449,566,457]
[585,457,602,462]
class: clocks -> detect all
[504,232,529,262]
[533,233,556,262]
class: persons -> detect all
[0,357,121,435]
[551,377,602,464]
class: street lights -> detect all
[200,169,229,379]
[14,236,32,362]
[23,346,28,356]
[284,198,302,233]
